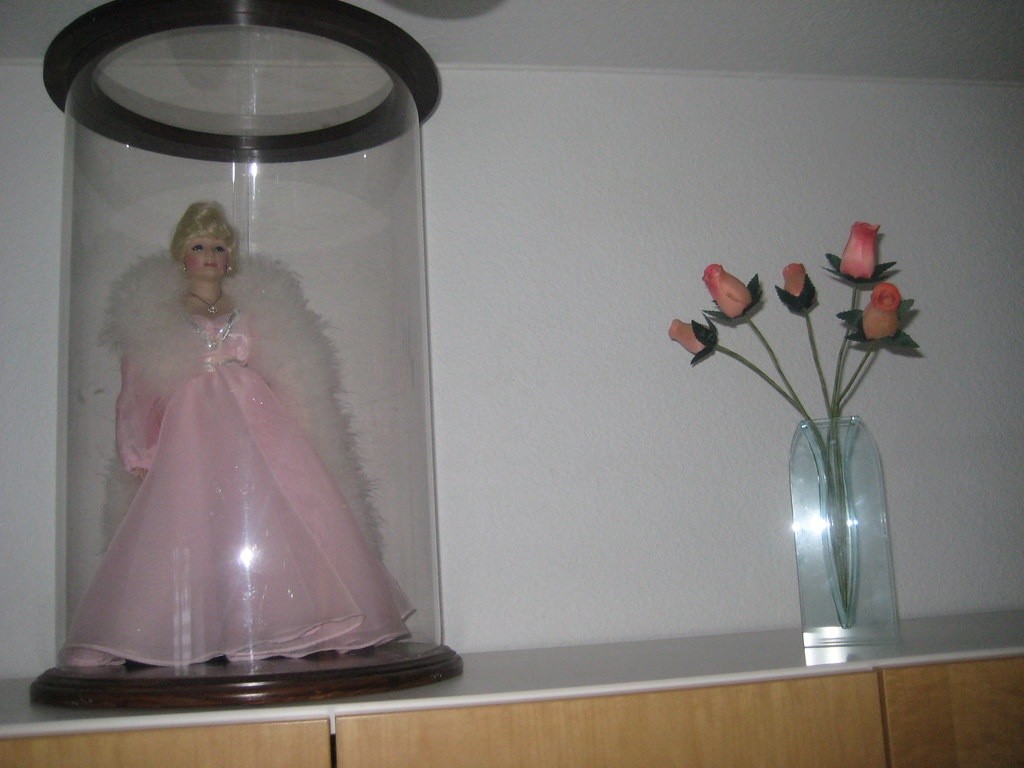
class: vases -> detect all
[787,415,904,651]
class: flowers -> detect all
[670,224,921,625]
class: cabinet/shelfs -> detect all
[0,611,1023,767]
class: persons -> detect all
[58,201,415,669]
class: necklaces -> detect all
[192,292,223,314]
